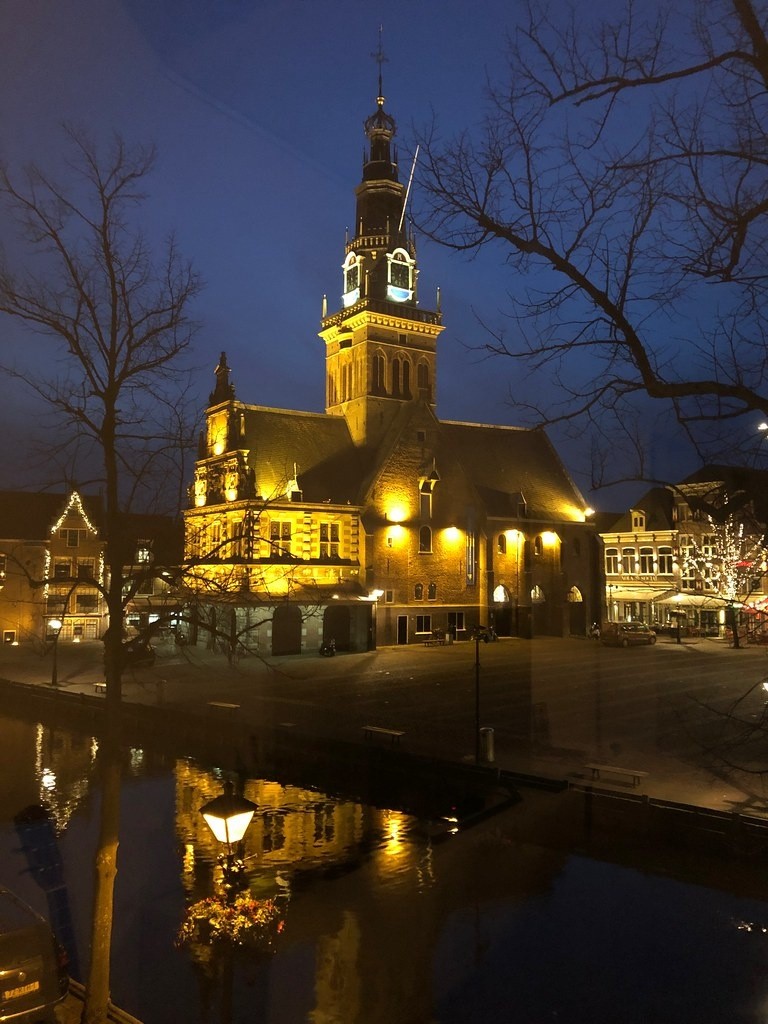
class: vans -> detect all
[603,621,657,647]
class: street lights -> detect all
[196,778,262,1024]
[44,616,65,688]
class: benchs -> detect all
[207,701,240,718]
[585,762,650,789]
[423,639,445,647]
[362,725,406,749]
[94,681,107,693]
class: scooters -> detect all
[587,622,600,639]
[317,634,336,656]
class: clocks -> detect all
[343,262,361,294]
[387,258,412,290]
